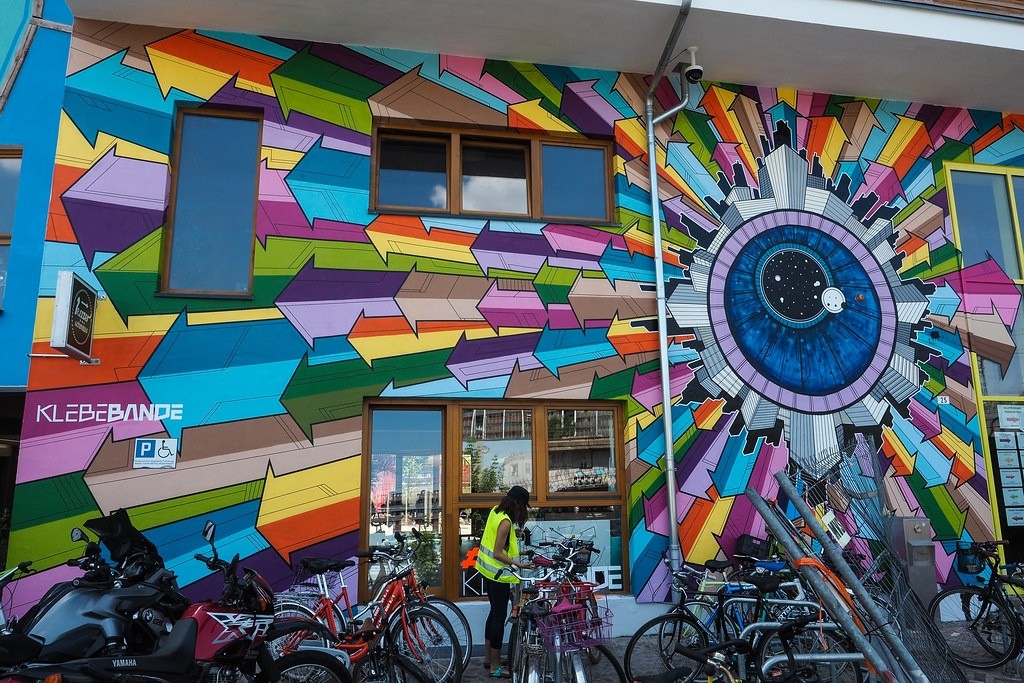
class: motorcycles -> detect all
[0,522,355,683]
[0,487,190,670]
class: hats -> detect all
[507,486,532,509]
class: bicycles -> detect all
[926,540,1024,672]
[223,508,905,682]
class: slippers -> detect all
[484,658,508,668]
[490,666,510,678]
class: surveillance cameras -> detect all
[685,65,703,83]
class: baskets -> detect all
[736,534,770,561]
[535,582,598,600]
[292,561,339,589]
[560,540,593,564]
[956,540,986,574]
[534,605,614,653]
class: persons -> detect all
[474,485,530,678]
[570,454,607,488]
